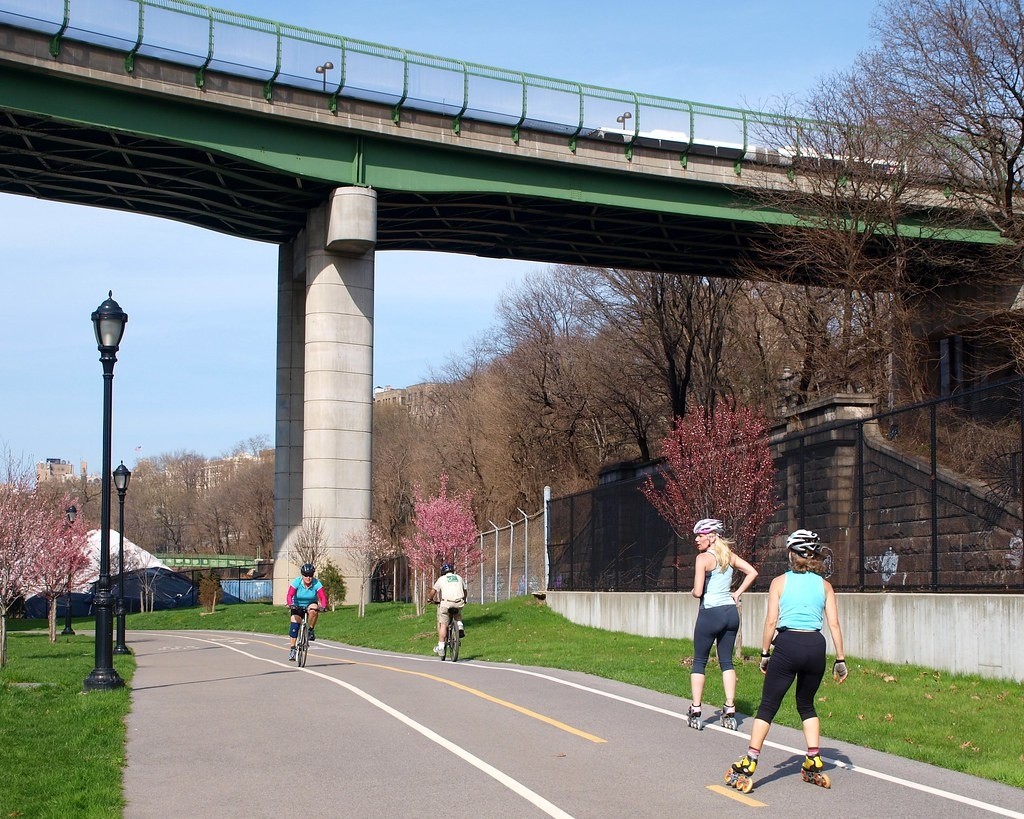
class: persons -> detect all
[286,564,328,662]
[724,530,848,793]
[684,519,758,731]
[427,564,467,656]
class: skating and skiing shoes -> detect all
[723,750,759,794]
[800,754,831,789]
[719,702,738,731]
[686,702,703,730]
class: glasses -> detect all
[303,574,313,577]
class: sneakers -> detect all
[289,648,296,661]
[457,624,465,638]
[308,629,315,641]
[433,645,446,656]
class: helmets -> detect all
[301,564,315,575]
[693,519,724,535]
[785,529,822,559]
[440,564,454,576]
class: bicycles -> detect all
[425,598,469,663]
[282,602,334,668]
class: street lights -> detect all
[81,289,133,688]
[314,62,337,102]
[112,460,132,657]
[61,504,77,635]
[615,112,634,135]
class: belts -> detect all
[441,599,461,603]
